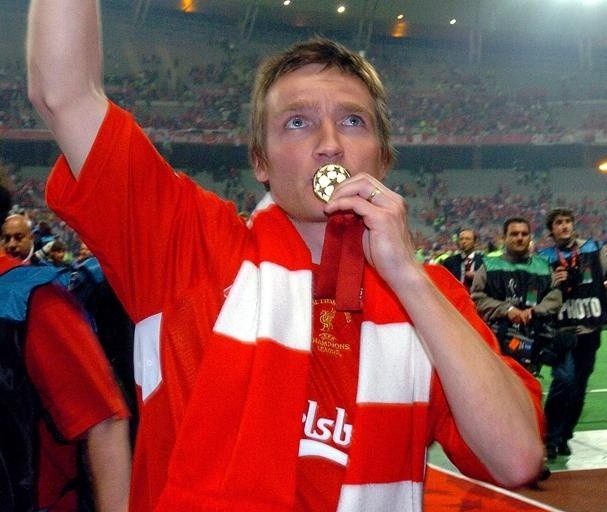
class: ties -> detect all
[463,259,473,288]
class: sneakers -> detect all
[536,466,550,479]
[545,442,557,461]
[559,442,570,455]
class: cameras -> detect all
[562,265,584,286]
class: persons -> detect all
[0,27,607,484]
[0,168,136,511]
[23,0,549,511]
